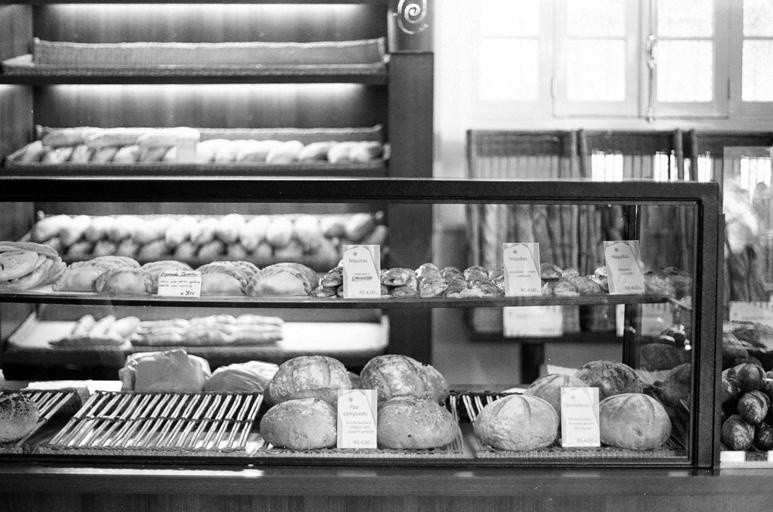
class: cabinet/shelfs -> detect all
[469,125,773,346]
[0,174,726,473]
[1,0,440,380]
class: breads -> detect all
[53,256,141,292]
[0,242,64,292]
[262,397,336,449]
[554,280,578,298]
[415,262,445,299]
[540,263,563,280]
[381,268,408,285]
[320,269,345,286]
[268,356,351,403]
[0,392,37,442]
[143,260,197,295]
[246,263,319,300]
[577,360,643,400]
[526,373,592,412]
[193,261,259,302]
[443,267,470,298]
[360,354,447,405]
[377,397,457,448]
[579,273,601,297]
[464,265,506,297]
[98,270,147,299]
[210,360,277,396]
[600,393,671,448]
[118,348,210,395]
[475,393,559,450]
[394,275,418,299]
